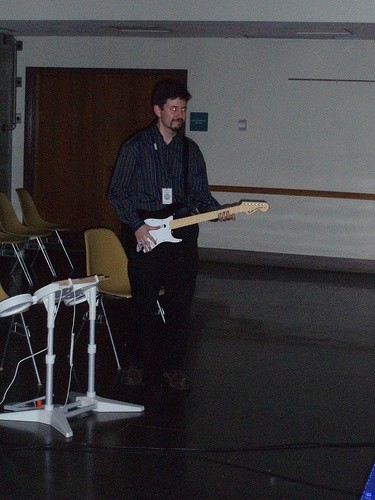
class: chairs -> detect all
[0,186,77,317]
[67,227,173,372]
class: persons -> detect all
[108,79,237,391]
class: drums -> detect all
[33,279,72,303]
[0,293,34,316]
[64,287,99,306]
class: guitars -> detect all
[136,200,270,253]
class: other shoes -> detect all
[161,358,192,392]
[117,360,149,391]
